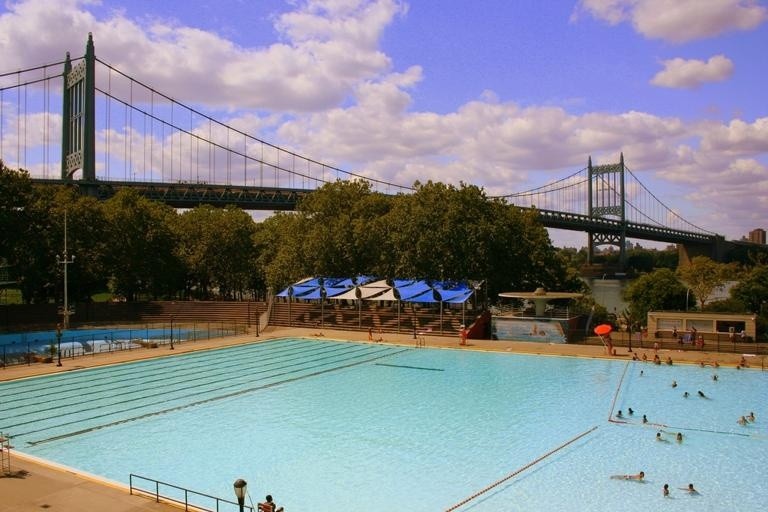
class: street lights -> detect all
[55,211,75,325]
[231,479,248,511]
[27,338,38,365]
[3,341,16,366]
[254,303,262,337]
[55,324,65,367]
[169,314,176,349]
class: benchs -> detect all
[0,299,480,337]
[513,307,574,318]
[584,329,768,355]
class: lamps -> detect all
[234,479,248,512]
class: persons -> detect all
[687,327,696,336]
[664,484,669,495]
[696,335,705,351]
[672,326,678,337]
[740,330,746,340]
[677,433,682,440]
[369,329,373,340]
[264,495,284,512]
[605,334,613,356]
[460,327,468,345]
[654,342,659,354]
[616,349,756,428]
[615,471,645,480]
[688,484,695,492]
[728,328,734,341]
[656,433,666,442]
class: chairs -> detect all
[257,503,285,512]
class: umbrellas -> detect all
[594,324,612,335]
